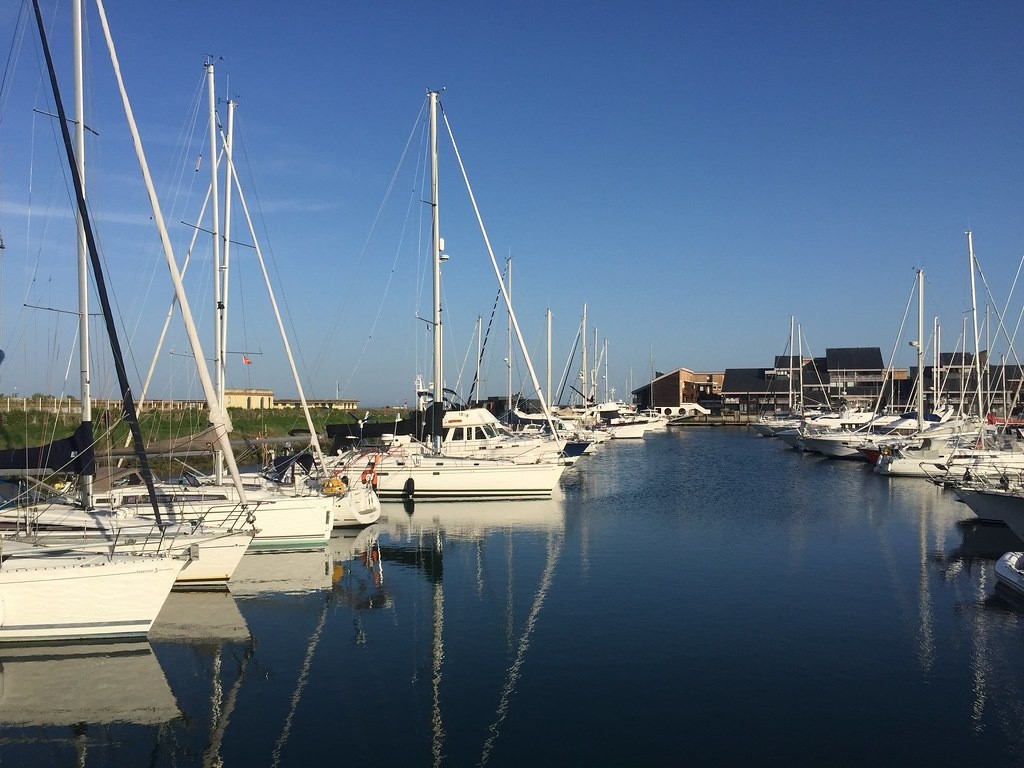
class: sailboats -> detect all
[146,584,254,768]
[0,0,671,642]
[377,498,563,768]
[0,639,190,768]
[197,544,333,768]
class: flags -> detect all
[244,354,253,364]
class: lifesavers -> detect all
[360,469,378,485]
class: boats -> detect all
[745,228,1024,544]
[993,551,1024,597]
